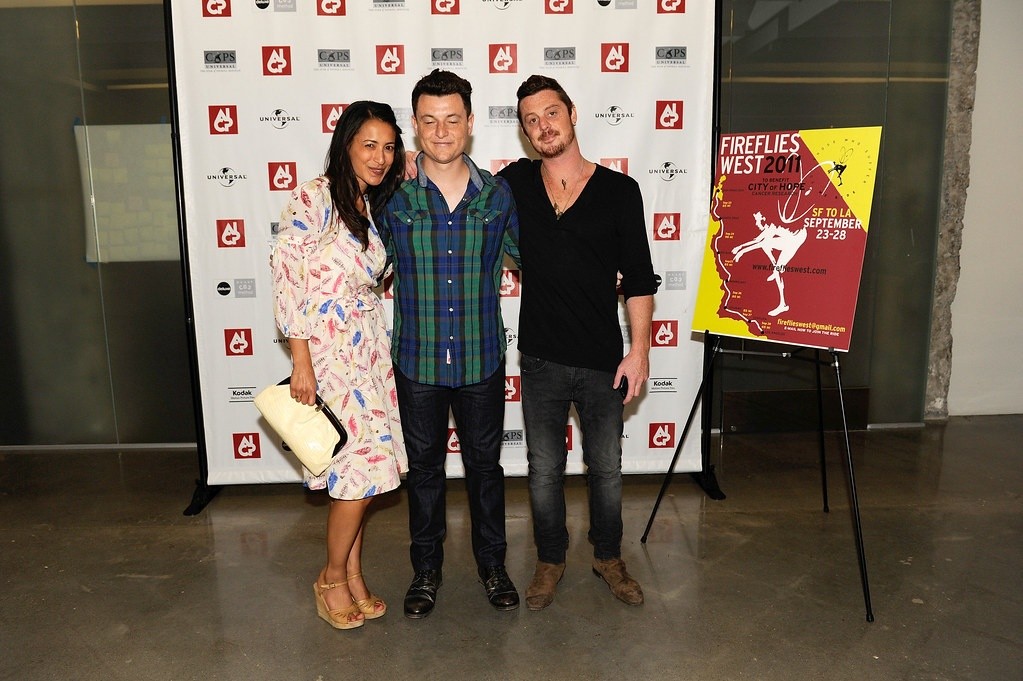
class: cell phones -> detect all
[619,376,628,398]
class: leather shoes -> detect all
[404,567,444,619]
[478,567,520,611]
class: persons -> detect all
[272,100,410,630]
[407,75,663,610]
[378,71,623,619]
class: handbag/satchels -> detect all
[253,376,349,478]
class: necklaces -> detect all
[542,158,583,220]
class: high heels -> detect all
[346,572,386,620]
[313,580,365,630]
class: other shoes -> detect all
[525,559,567,611]
[591,555,645,606]
